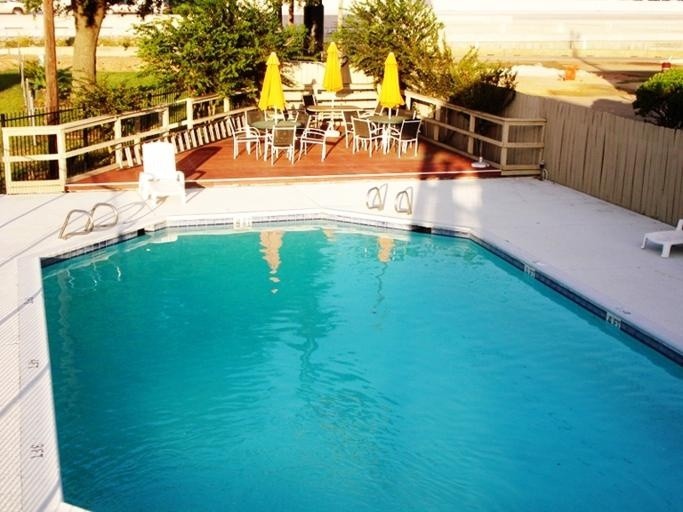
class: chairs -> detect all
[137,141,186,211]
[640,220,682,259]
[226,94,422,166]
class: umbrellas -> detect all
[259,230,285,275]
[321,41,344,131]
[257,52,285,126]
[376,51,406,152]
